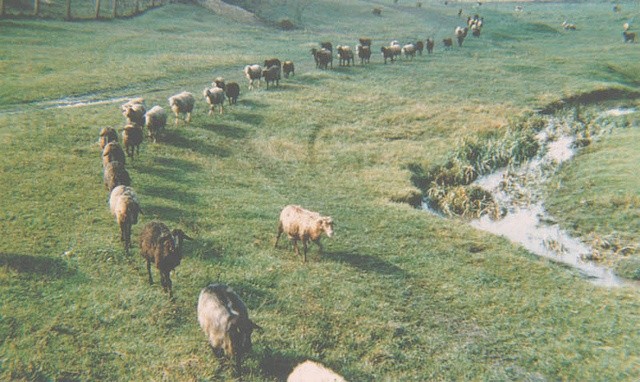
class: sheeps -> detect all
[286,361,345,382]
[245,64,262,89]
[143,105,167,142]
[140,222,193,298]
[274,205,336,261]
[264,59,281,68]
[118,122,143,160]
[127,105,145,126]
[169,91,195,125]
[336,45,354,66]
[358,45,369,64]
[204,87,225,115]
[197,284,261,375]
[103,161,130,190]
[109,186,143,256]
[262,65,281,87]
[372,0,635,63]
[283,61,295,77]
[311,48,326,67]
[223,82,239,104]
[99,126,117,149]
[214,77,226,90]
[359,38,371,50]
[319,41,332,53]
[316,50,333,69]
[102,142,125,165]
